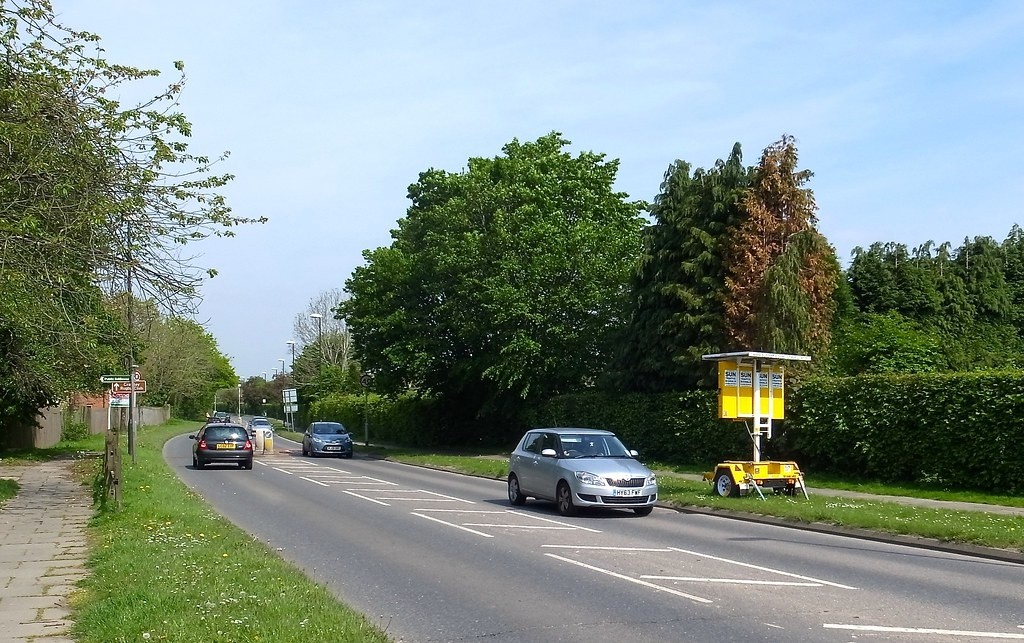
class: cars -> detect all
[249,416,276,437]
[206,410,231,424]
[301,421,354,458]
[506,427,659,517]
[188,422,254,470]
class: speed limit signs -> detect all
[134,371,142,381]
[360,375,373,387]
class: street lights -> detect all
[271,368,278,422]
[310,313,323,422]
[285,341,295,431]
[261,371,266,412]
[278,359,287,423]
[237,382,242,420]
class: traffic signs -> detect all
[112,380,147,395]
[100,374,130,384]
